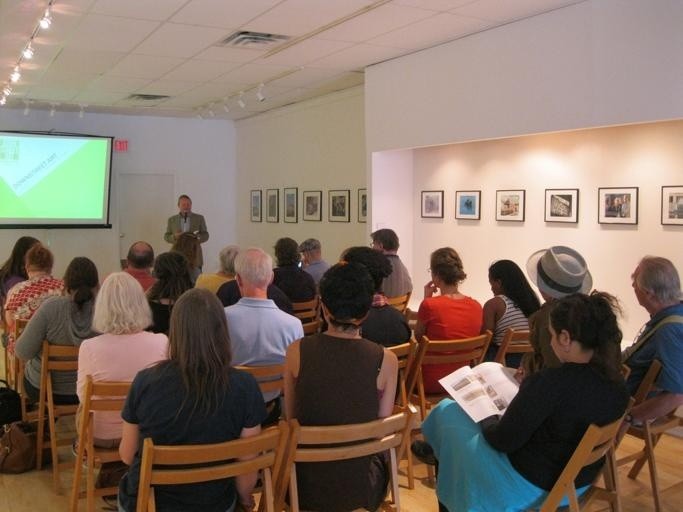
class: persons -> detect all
[162,193,209,273]
[612,255,682,429]
[410,248,484,400]
[517,244,594,378]
[408,285,634,512]
[0,230,401,510]
[482,259,545,370]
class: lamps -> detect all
[191,66,303,121]
[0,0,55,108]
[16,98,90,119]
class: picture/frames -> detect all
[421,185,683,225]
[249,187,367,224]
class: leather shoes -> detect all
[411,440,437,465]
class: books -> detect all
[439,361,523,424]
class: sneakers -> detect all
[73,440,100,469]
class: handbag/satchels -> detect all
[0,417,59,474]
[1,379,22,424]
[96,461,130,500]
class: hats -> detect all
[526,246,592,298]
[298,239,321,251]
